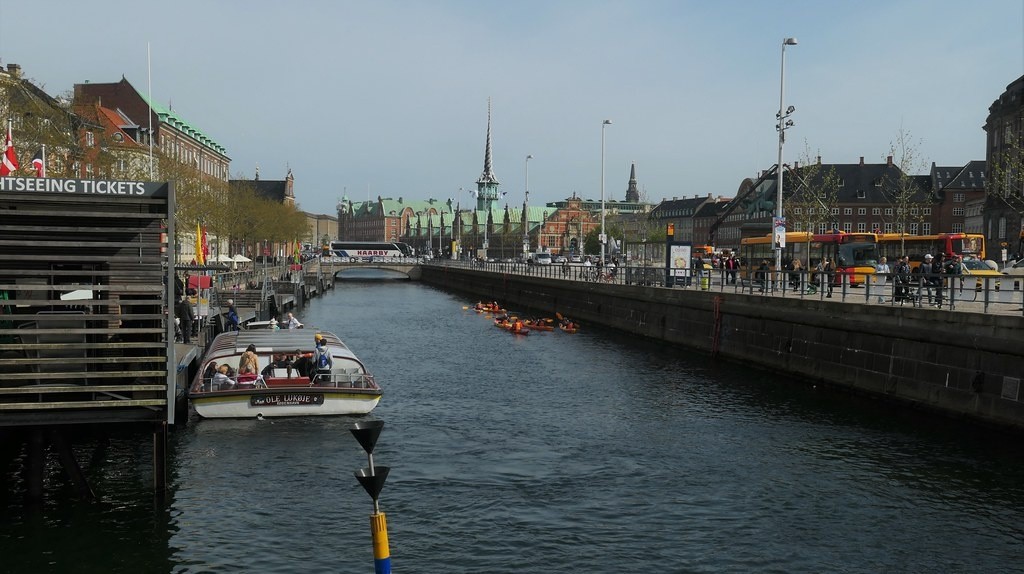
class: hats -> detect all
[315,334,322,341]
[219,367,226,372]
[925,254,934,259]
[952,255,959,260]
[269,319,276,324]
[942,253,947,256]
[246,365,252,371]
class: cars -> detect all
[301,252,321,263]
[417,255,432,265]
[487,257,524,262]
[941,259,1006,275]
[1000,260,1024,274]
[554,254,612,264]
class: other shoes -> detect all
[880,300,885,304]
[187,342,193,344]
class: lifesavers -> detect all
[304,353,314,358]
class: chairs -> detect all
[205,366,368,391]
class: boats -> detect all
[473,307,506,314]
[494,317,531,336]
[559,322,577,334]
[187,320,387,418]
[525,324,555,331]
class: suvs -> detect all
[256,256,272,263]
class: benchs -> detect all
[896,276,917,308]
[740,275,768,295]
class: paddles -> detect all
[558,313,579,328]
[483,307,506,310]
[556,312,563,318]
[525,319,553,323]
[462,306,475,309]
[542,318,549,321]
[485,316,517,320]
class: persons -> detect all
[239,344,259,375]
[876,256,911,304]
[225,299,238,332]
[268,318,279,329]
[786,256,805,291]
[178,296,195,343]
[814,257,836,298]
[607,257,619,275]
[584,258,603,282]
[288,313,300,329]
[758,260,769,292]
[312,334,333,374]
[471,255,484,267]
[527,256,533,271]
[913,252,970,309]
[775,220,785,248]
[203,362,235,389]
[695,249,740,284]
[261,349,312,378]
[562,258,569,279]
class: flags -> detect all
[196,222,208,265]
[31,150,43,177]
[293,240,301,264]
[0,129,19,177]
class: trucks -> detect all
[692,246,724,259]
[527,253,552,266]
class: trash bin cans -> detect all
[701,270,713,287]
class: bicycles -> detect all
[580,269,620,285]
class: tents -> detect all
[209,254,251,271]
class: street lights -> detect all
[457,188,463,260]
[774,37,797,289]
[263,239,268,265]
[242,238,245,267]
[600,120,613,282]
[524,155,533,263]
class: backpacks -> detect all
[318,348,329,368]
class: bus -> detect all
[876,232,986,281]
[740,231,879,287]
[322,246,330,263]
[329,241,416,264]
[303,242,312,253]
